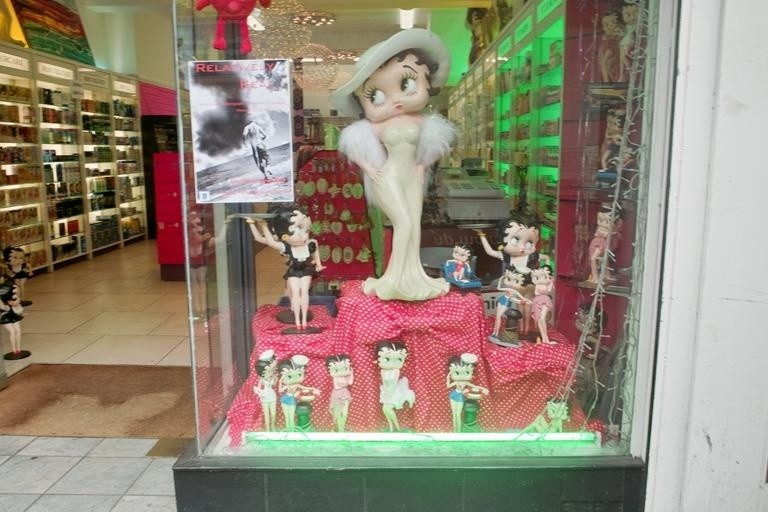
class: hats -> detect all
[329,27,452,120]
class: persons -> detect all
[372,340,417,433]
[529,263,555,344]
[572,1,643,370]
[444,353,493,431]
[327,25,460,302]
[254,206,329,331]
[489,263,532,339]
[0,282,24,356]
[0,245,35,302]
[275,352,319,431]
[324,353,356,433]
[252,347,280,432]
[472,215,551,337]
[517,395,571,431]
[243,120,273,181]
[240,203,305,317]
[441,241,483,291]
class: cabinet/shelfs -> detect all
[0,40,149,274]
[446,1,649,428]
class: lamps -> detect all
[328,50,360,61]
[399,7,416,29]
[290,11,336,27]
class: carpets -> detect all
[0,364,221,440]
